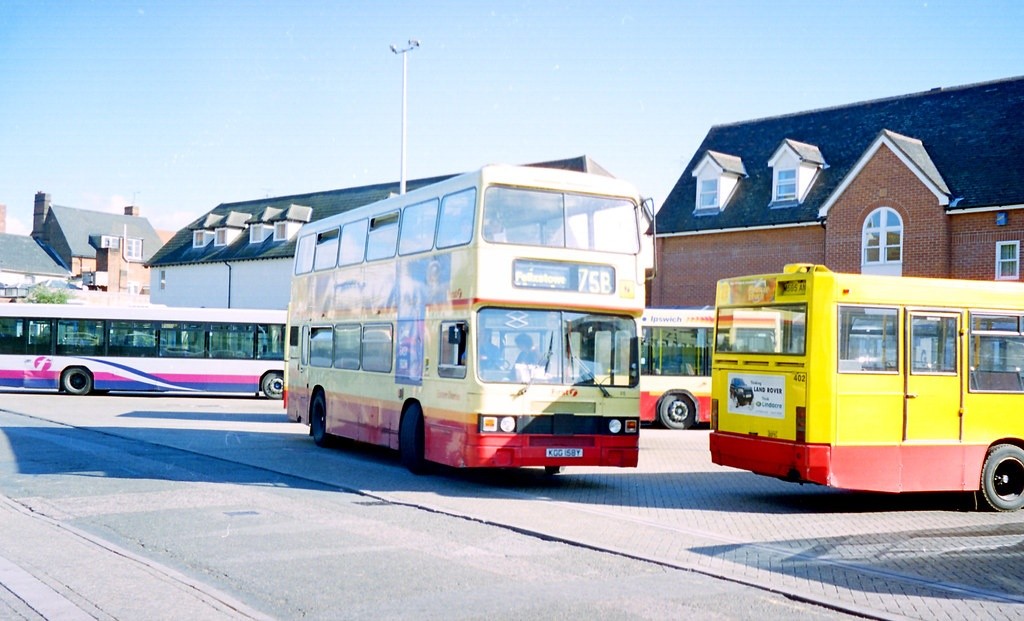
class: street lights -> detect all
[389,37,420,193]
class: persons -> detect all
[460,327,501,370]
[509,334,536,381]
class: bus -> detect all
[709,262,1024,511]
[282,164,658,477]
[0,300,287,400]
[311,329,391,373]
[594,309,781,431]
